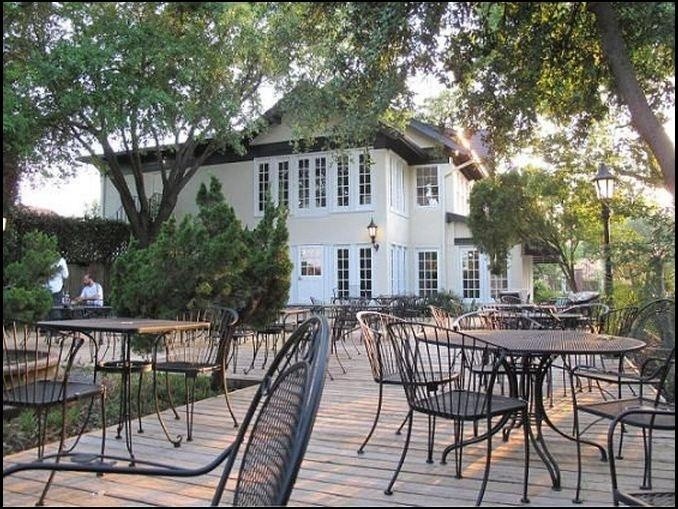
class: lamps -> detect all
[367,218,378,251]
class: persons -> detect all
[73,272,106,320]
[40,252,72,320]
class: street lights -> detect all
[589,163,616,335]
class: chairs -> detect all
[569,300,677,503]
[1,316,106,506]
[137,305,238,441]
[213,295,636,406]
[2,316,332,508]
[452,310,550,439]
[385,322,529,507]
[355,311,459,464]
[609,407,677,507]
